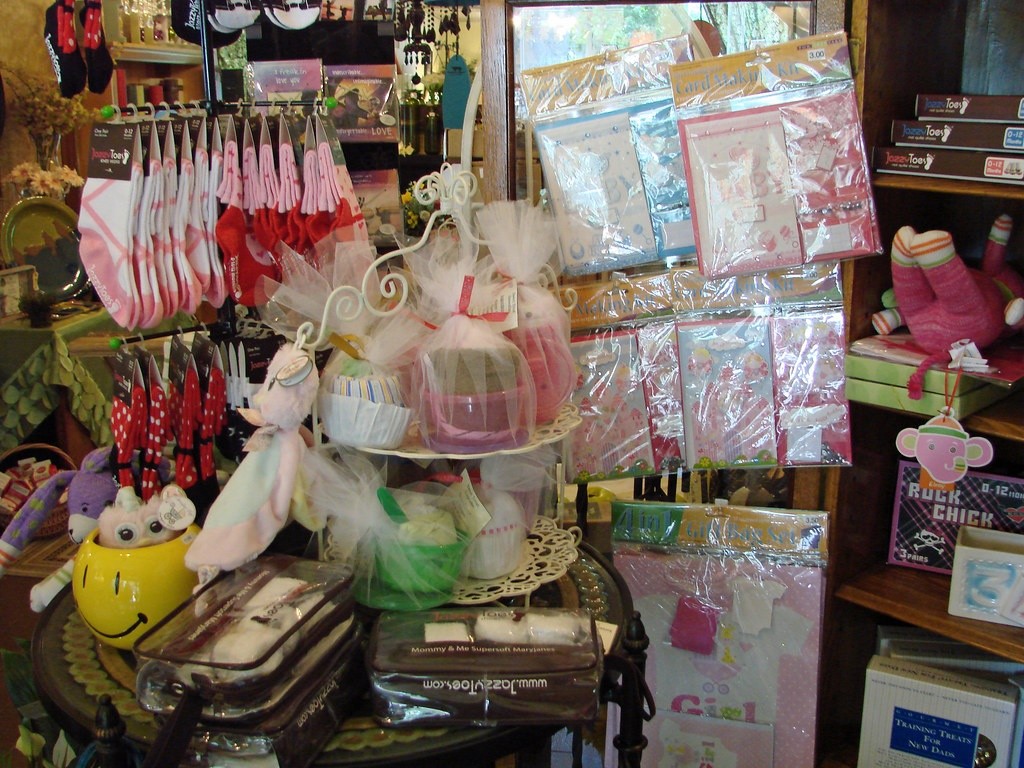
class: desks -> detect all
[32,529,656,768]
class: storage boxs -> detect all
[888,460,1024,575]
[947,525,1024,628]
[845,376,1006,421]
[843,352,990,397]
[890,119,1024,155]
[889,640,1024,683]
[915,92,1024,125]
[870,146,1024,185]
[855,655,1019,768]
[876,625,971,656]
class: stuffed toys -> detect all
[0,331,322,609]
[870,213,1023,401]
